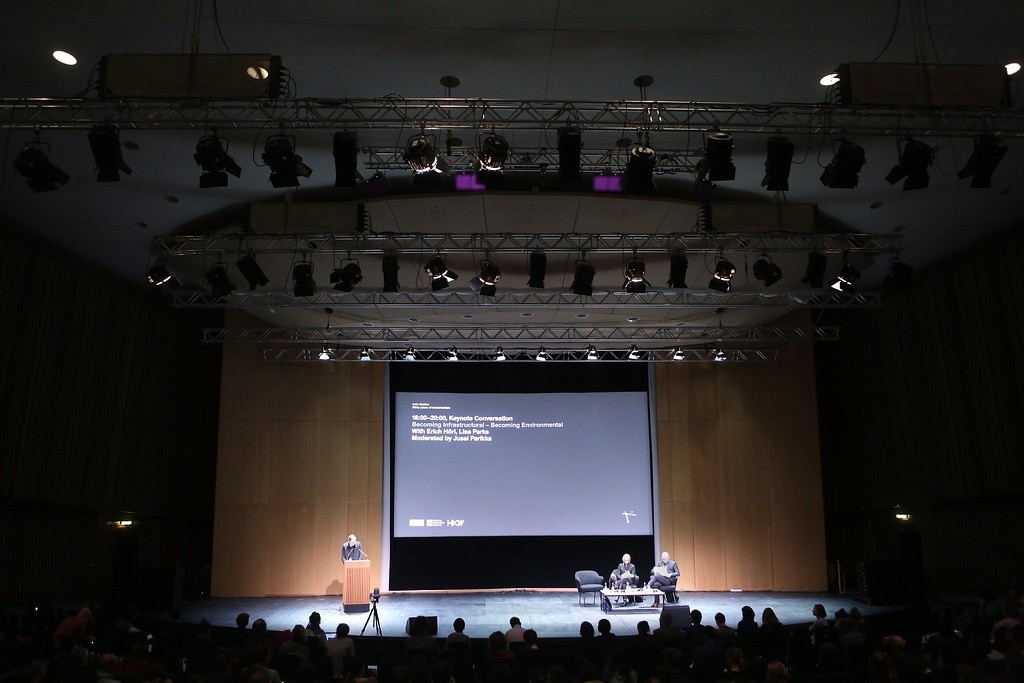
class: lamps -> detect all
[331,130,364,190]
[569,259,594,296]
[829,264,860,294]
[710,262,737,293]
[328,258,363,293]
[193,135,242,189]
[956,133,1010,190]
[695,130,736,183]
[13,139,70,192]
[261,134,313,188]
[204,262,237,299]
[761,136,794,192]
[619,145,659,198]
[666,254,687,289]
[467,261,501,297]
[424,259,459,290]
[525,251,548,288]
[402,134,452,179]
[885,137,936,191]
[476,133,509,175]
[820,138,865,189]
[292,259,318,299]
[799,252,829,289]
[236,254,269,294]
[553,128,585,178]
[754,256,783,287]
[88,124,133,183]
[622,256,652,295]
[878,261,916,302]
[381,254,399,293]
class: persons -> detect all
[643,551,681,608]
[0,593,1024,683]
[614,553,635,607]
[341,534,363,564]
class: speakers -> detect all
[406,616,438,635]
[660,605,690,632]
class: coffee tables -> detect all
[599,588,665,615]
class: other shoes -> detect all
[614,599,618,603]
[620,602,627,606]
[651,602,660,608]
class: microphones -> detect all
[346,549,353,560]
[359,549,368,559]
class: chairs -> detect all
[658,585,676,608]
[574,570,604,606]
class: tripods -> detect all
[360,602,382,636]
